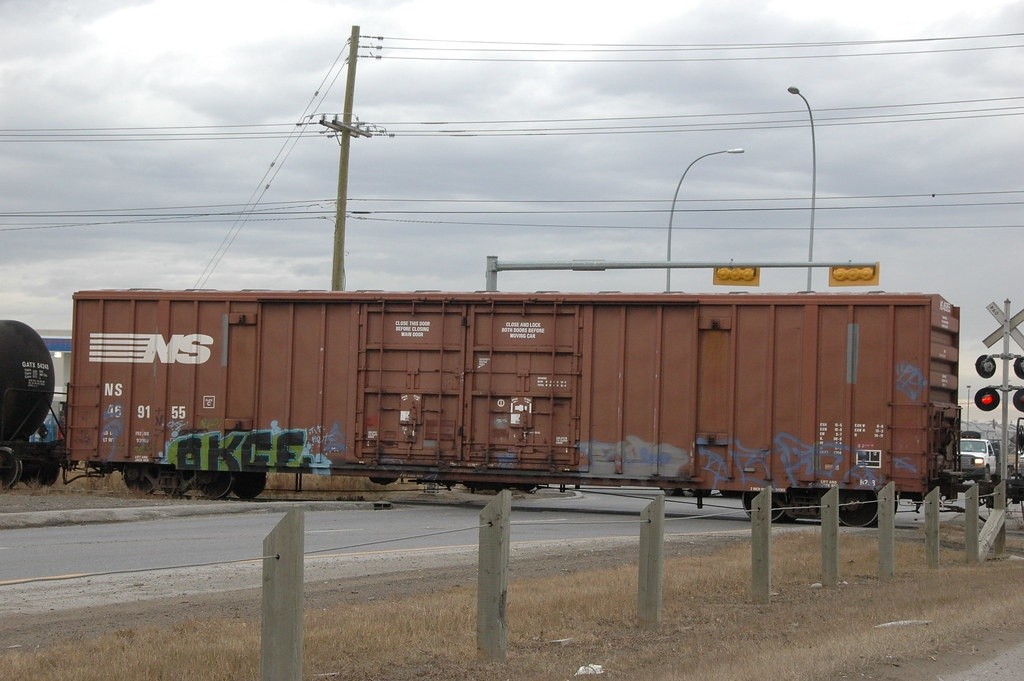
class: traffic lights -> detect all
[974,388,1000,411]
[1013,389,1024,412]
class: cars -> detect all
[1019,457,1024,463]
[957,439,997,483]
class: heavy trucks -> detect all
[0,289,972,527]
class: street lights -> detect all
[967,385,970,431]
[666,149,744,292]
[788,87,816,291]
